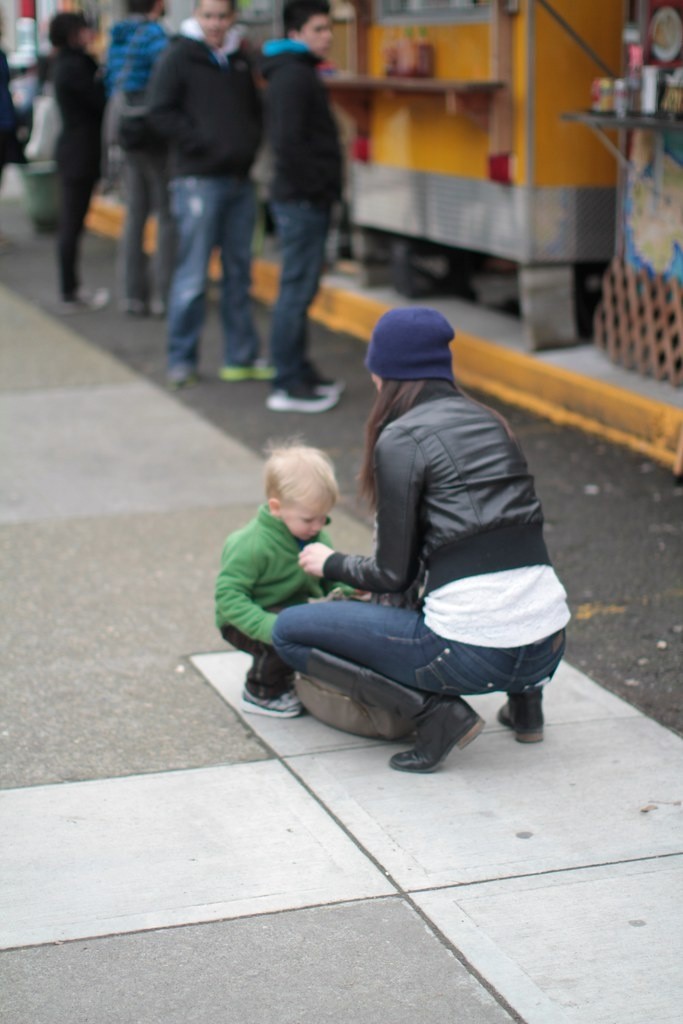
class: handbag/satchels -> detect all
[294,586,417,740]
[24,69,64,159]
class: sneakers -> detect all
[242,688,303,718]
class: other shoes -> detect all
[60,288,111,315]
[266,379,346,413]
[125,298,167,316]
[219,357,278,382]
[169,374,201,390]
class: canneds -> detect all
[592,77,637,118]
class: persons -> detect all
[0,50,28,175]
[270,307,572,772]
[216,443,371,718]
[146,0,278,388]
[49,12,111,316]
[254,0,348,413]
[103,0,177,320]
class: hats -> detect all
[48,12,86,46]
[365,306,454,382]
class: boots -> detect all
[497,685,544,743]
[307,646,485,773]
